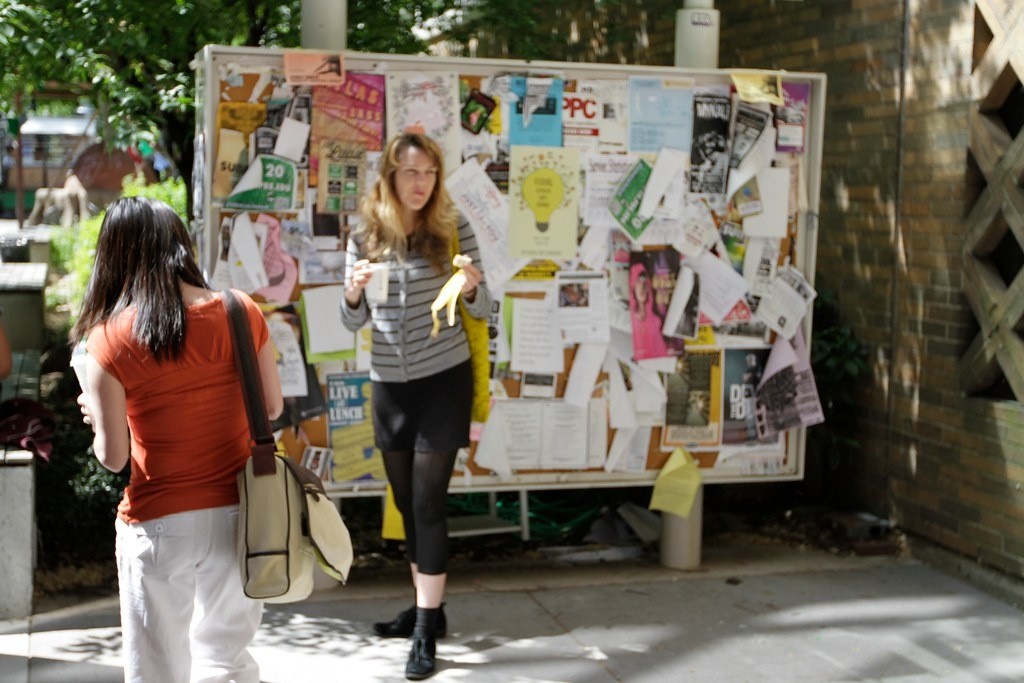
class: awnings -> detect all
[19,118,97,137]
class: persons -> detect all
[630,264,667,357]
[338,133,492,678]
[561,285,587,306]
[71,198,282,683]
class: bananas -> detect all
[453,254,472,269]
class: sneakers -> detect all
[373,603,447,638]
[405,636,437,678]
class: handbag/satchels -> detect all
[221,288,353,603]
